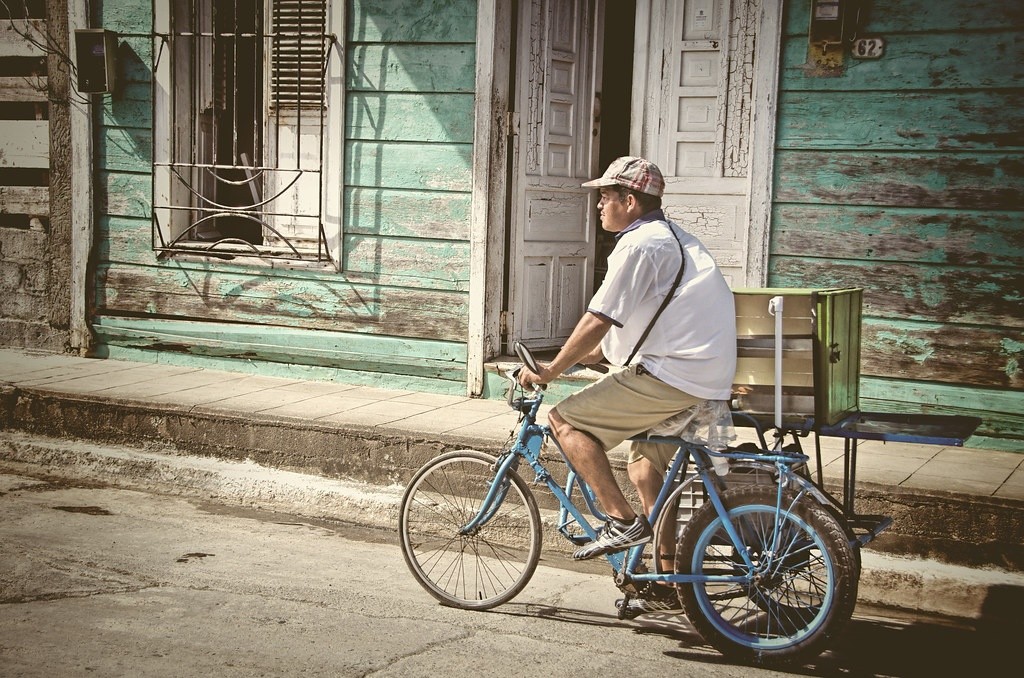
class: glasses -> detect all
[602,196,622,205]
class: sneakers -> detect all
[571,513,654,561]
[615,581,685,615]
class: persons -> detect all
[519,157,737,616]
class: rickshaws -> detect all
[399,341,979,669]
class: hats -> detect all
[581,156,665,197]
